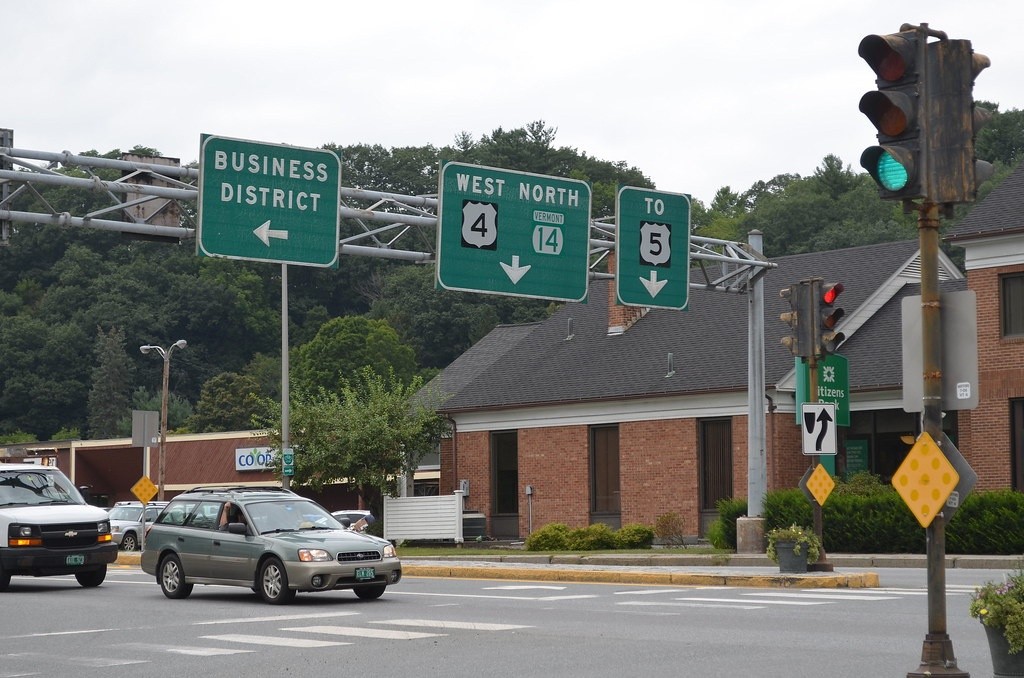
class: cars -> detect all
[1,462,119,588]
[107,500,219,552]
[138,483,403,601]
[314,509,372,535]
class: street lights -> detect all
[141,340,188,500]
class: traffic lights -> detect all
[859,26,929,205]
[926,40,994,202]
[778,288,798,359]
[812,280,846,354]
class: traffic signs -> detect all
[201,138,345,267]
